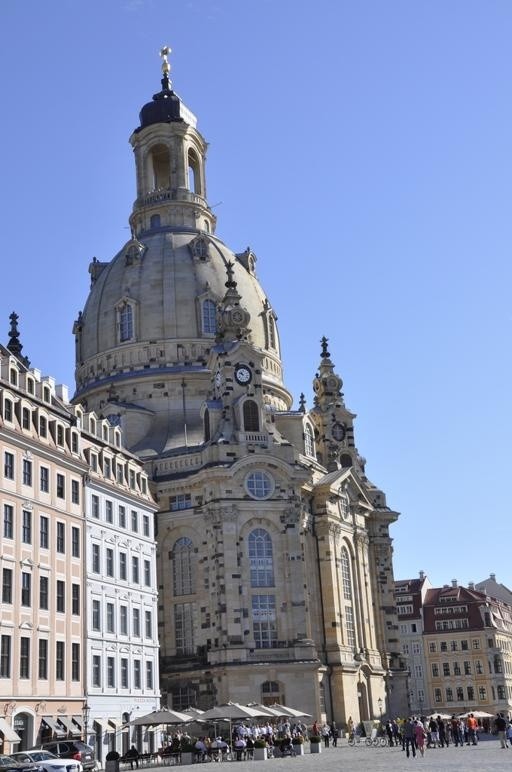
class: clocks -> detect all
[234,364,252,387]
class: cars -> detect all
[8,750,83,772]
[0,753,39,772]
[27,739,96,772]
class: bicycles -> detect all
[348,728,390,748]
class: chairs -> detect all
[197,745,254,763]
[121,749,179,770]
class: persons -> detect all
[494,713,511,748]
[173,732,228,761]
[322,721,338,747]
[348,717,356,742]
[232,719,307,761]
[128,745,139,770]
[386,713,479,758]
[478,718,490,733]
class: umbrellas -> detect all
[126,700,314,748]
[457,710,495,720]
[426,710,452,720]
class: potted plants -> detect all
[104,751,120,772]
[180,743,197,765]
[253,734,322,761]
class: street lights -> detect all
[81,704,91,744]
[377,697,384,728]
